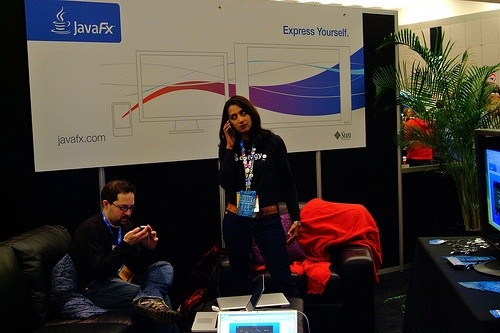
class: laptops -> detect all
[190,274,265,332]
[216,293,291,312]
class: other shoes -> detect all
[132,298,179,323]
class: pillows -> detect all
[50,253,109,318]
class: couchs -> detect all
[0,219,136,333]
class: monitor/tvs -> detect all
[218,309,298,333]
[472,128,500,278]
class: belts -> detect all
[226,202,278,218]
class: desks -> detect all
[176,270,307,333]
[405,234,500,333]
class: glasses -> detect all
[113,204,136,212]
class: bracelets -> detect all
[294,221,301,227]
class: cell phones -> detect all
[227,122,232,132]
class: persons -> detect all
[75,180,182,333]
[219,96,302,298]
[401,82,500,167]
